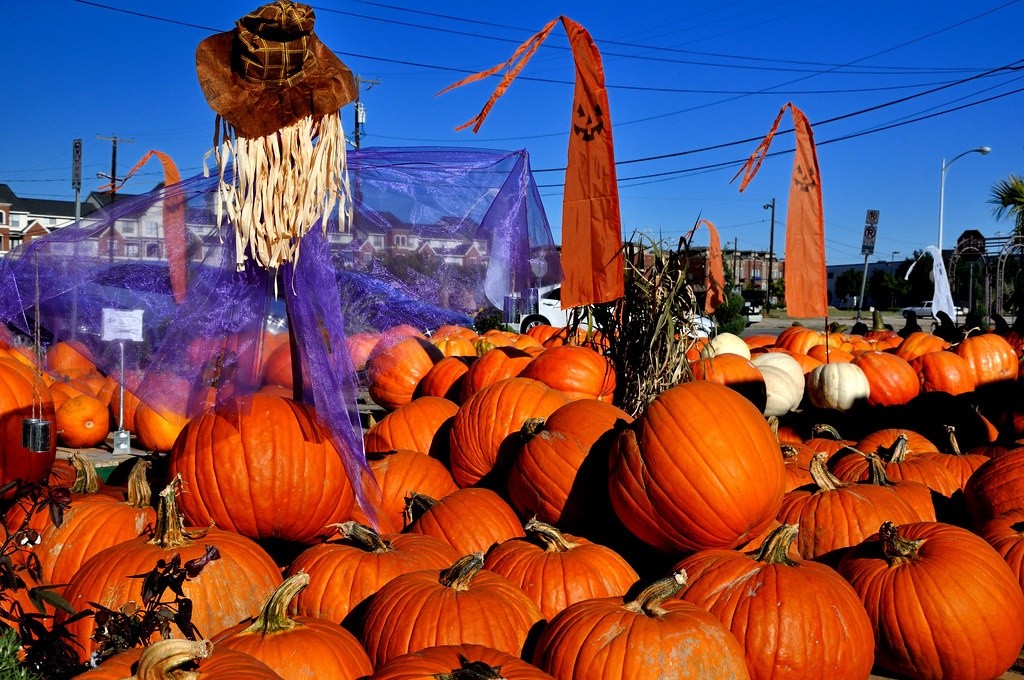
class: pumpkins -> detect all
[0,322,1024,680]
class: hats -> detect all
[196,0,358,139]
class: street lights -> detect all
[96,172,123,264]
[891,251,899,309]
[763,197,776,313]
[938,146,991,255]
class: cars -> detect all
[745,306,763,328]
[902,301,936,320]
[504,282,719,340]
